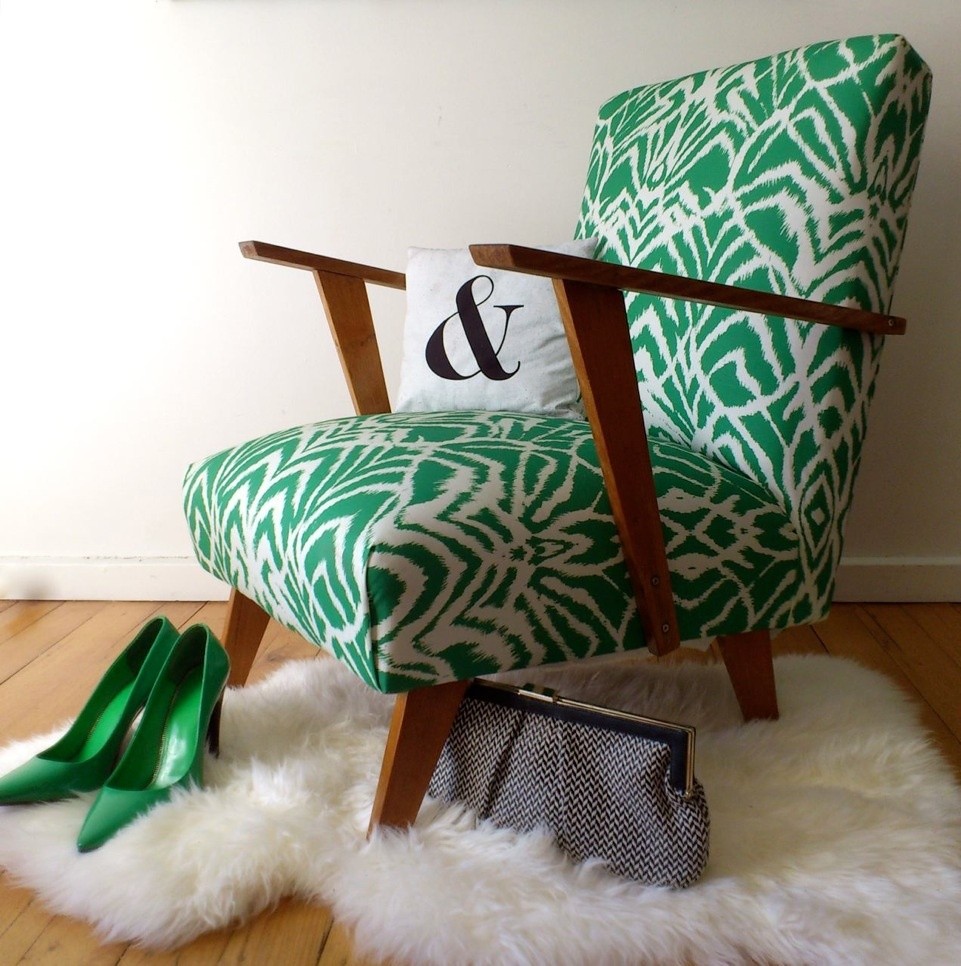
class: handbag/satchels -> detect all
[427,677,711,891]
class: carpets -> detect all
[0,649,961,966]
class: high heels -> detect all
[77,623,229,851]
[0,616,181,807]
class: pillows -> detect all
[397,239,599,418]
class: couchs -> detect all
[182,32,935,841]
